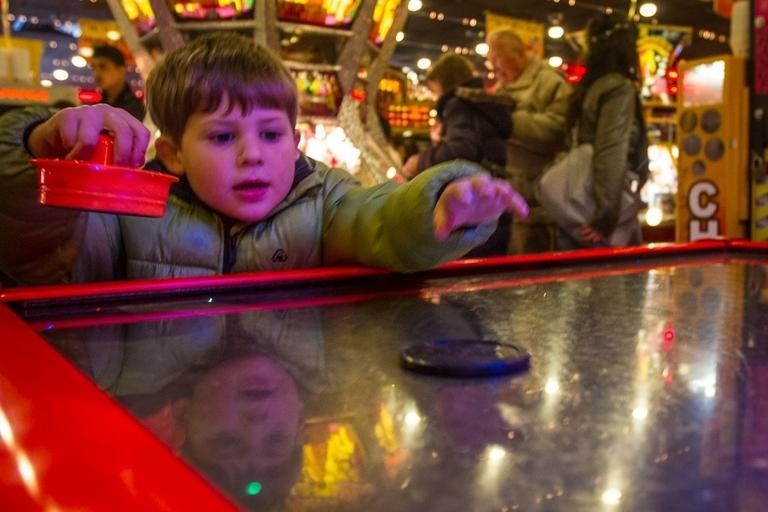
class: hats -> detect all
[417,54,474,96]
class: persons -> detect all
[546,11,650,254]
[399,49,518,259]
[42,295,524,509]
[487,25,582,255]
[88,44,147,122]
[1,32,531,276]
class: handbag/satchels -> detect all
[533,142,644,250]
